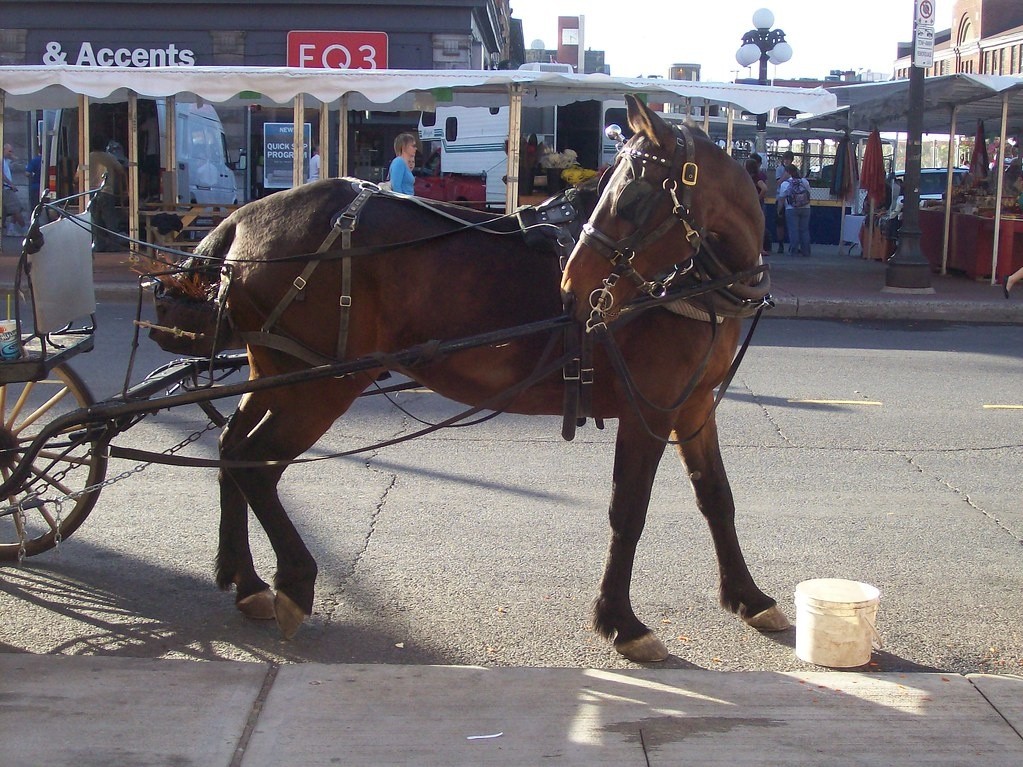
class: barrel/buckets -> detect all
[794,578,883,668]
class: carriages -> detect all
[0,87,791,660]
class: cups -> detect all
[0,320,22,360]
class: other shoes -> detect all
[6,226,17,237]
[799,251,810,257]
[107,244,116,251]
[1003,275,1009,299]
[762,250,772,255]
[20,225,32,237]
[790,250,798,257]
[96,243,105,251]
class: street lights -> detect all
[736,7,793,175]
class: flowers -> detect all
[518,133,598,185]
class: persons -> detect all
[744,150,813,256]
[306,145,320,183]
[1002,266,1023,299]
[138,111,159,202]
[73,133,125,250]
[387,133,419,197]
[3,143,42,236]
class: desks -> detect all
[843,214,865,256]
[143,201,245,254]
[915,210,1023,286]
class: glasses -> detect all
[409,143,417,148]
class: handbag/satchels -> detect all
[377,180,393,191]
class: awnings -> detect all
[789,72,1023,286]
[0,67,836,260]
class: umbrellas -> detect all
[968,118,989,181]
[860,131,884,257]
[828,132,860,256]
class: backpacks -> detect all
[785,177,810,207]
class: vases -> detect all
[546,166,567,190]
[519,167,536,196]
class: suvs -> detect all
[885,166,971,201]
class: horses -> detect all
[168,91,793,661]
